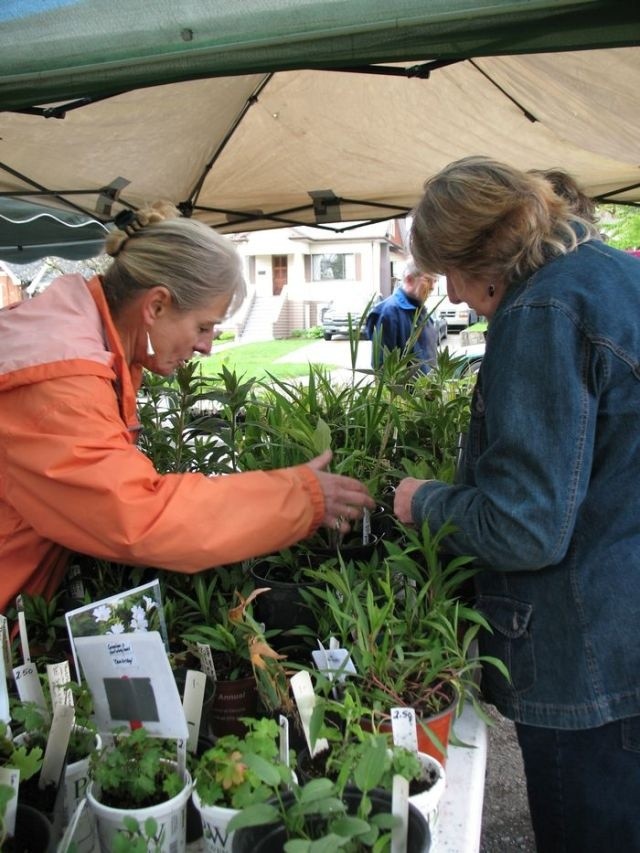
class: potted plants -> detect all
[0,274,515,853]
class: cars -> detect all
[321,290,384,342]
[445,341,487,392]
[423,303,448,346]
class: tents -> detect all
[1,0,637,259]
[0,196,112,265]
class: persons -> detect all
[1,204,376,649]
[364,255,438,375]
[390,154,639,852]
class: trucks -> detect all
[388,253,483,332]
